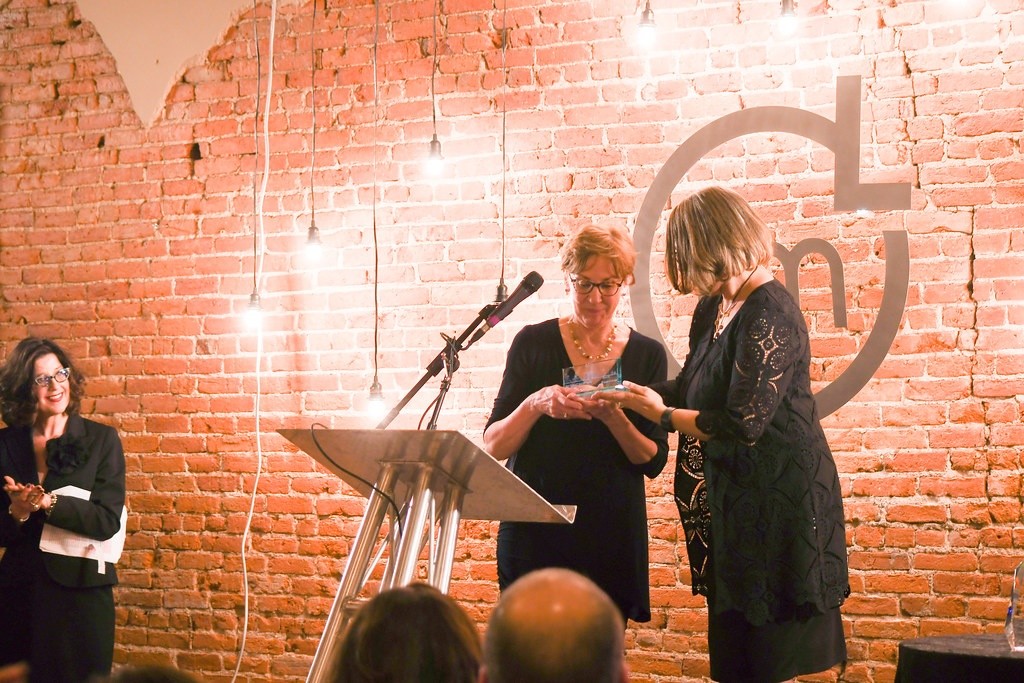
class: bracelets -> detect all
[45,492,57,515]
[660,407,676,433]
[9,504,30,522]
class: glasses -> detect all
[32,368,70,387]
[570,276,624,296]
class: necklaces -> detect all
[713,262,759,339]
[568,316,617,359]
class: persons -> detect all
[482,226,668,635]
[590,186,852,683]
[0,336,126,683]
[478,568,631,683]
[317,581,483,682]
[99,665,202,683]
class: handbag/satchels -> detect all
[39,484,127,564]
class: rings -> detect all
[563,413,567,418]
[30,502,40,511]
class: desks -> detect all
[894,634,1024,683]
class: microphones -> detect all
[469,271,543,345]
